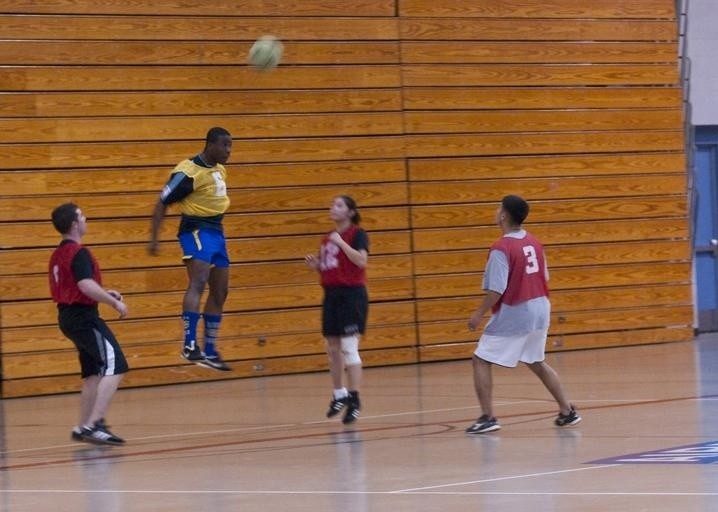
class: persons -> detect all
[301,193,371,429]
[147,126,237,372]
[45,202,135,448]
[458,193,584,438]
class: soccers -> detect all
[252,37,282,71]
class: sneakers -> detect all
[341,401,362,425]
[71,418,127,445]
[180,342,207,363]
[326,396,348,418]
[552,405,582,427]
[196,349,232,372]
[464,413,502,434]
